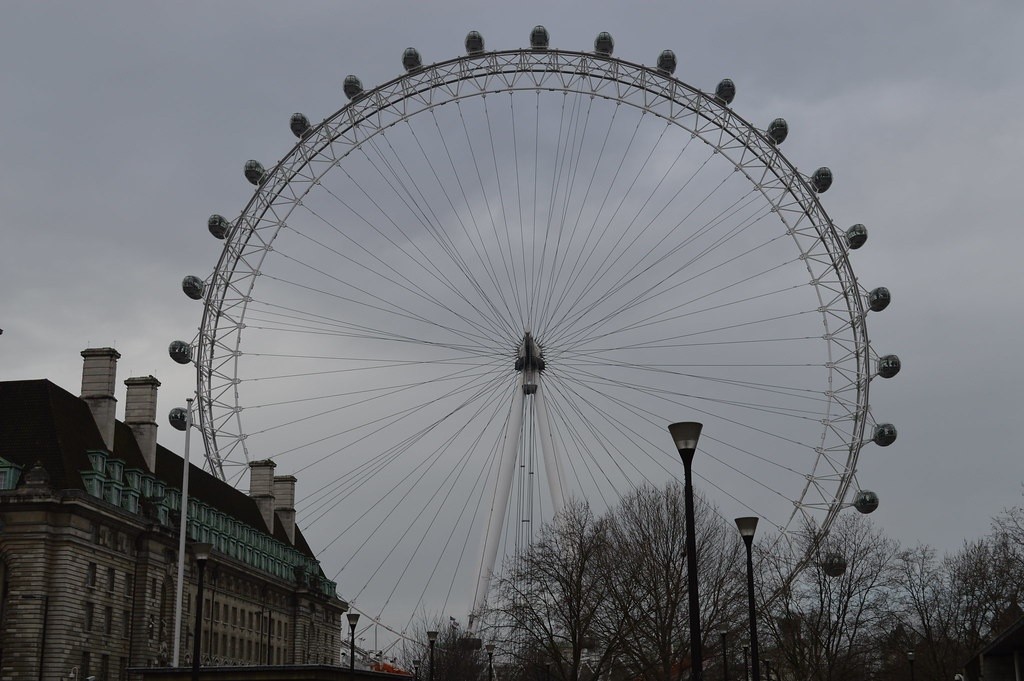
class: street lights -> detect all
[427,630,439,681]
[718,623,729,681]
[733,516,760,681]
[669,421,703,681]
[346,614,361,681]
[906,648,916,681]
[485,645,497,681]
